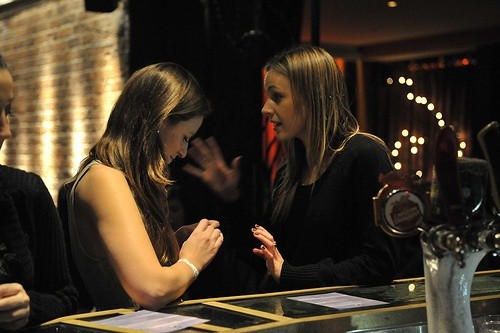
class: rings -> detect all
[274,241,277,246]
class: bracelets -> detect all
[177,258,200,278]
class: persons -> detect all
[183,44,397,290]
[0,54,81,333]
[58,61,224,311]
